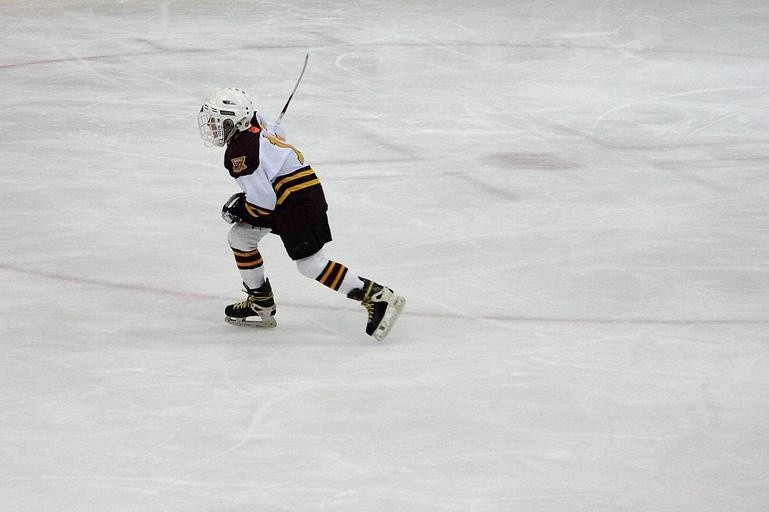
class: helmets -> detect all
[197,87,254,148]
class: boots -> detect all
[224,277,276,319]
[347,276,393,337]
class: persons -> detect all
[198,85,399,340]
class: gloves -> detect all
[227,197,246,224]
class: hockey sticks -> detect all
[219,49,309,223]
